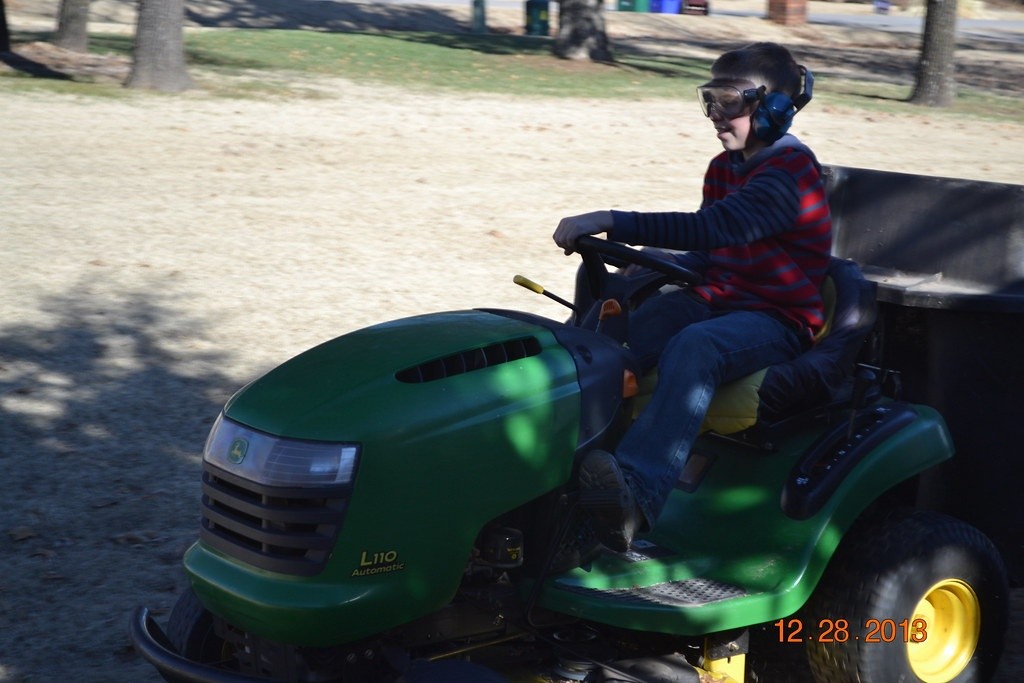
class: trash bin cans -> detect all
[524,0,549,36]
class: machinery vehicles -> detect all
[130,162,1024,683]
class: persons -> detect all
[553,41,835,553]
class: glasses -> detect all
[696,78,766,118]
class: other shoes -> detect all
[579,450,641,552]
